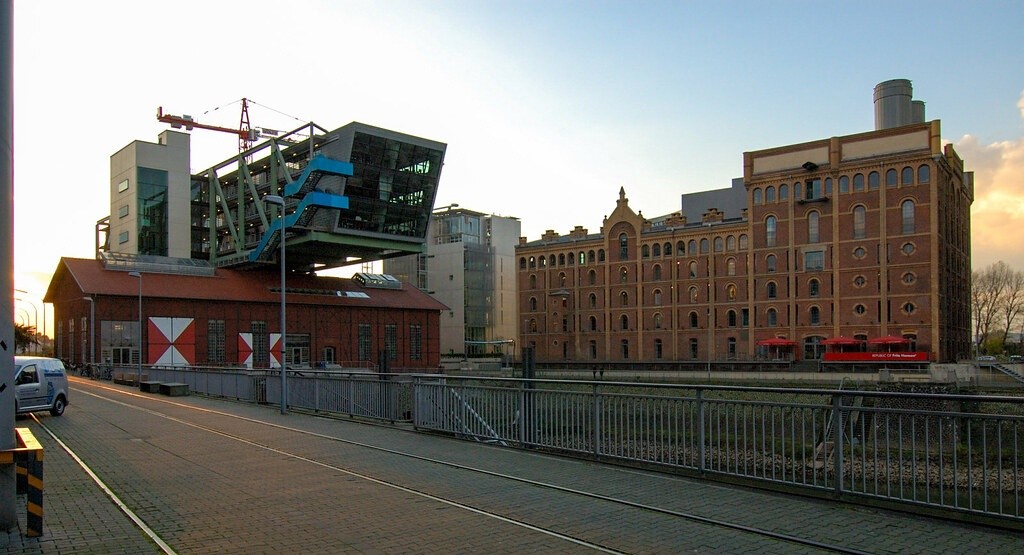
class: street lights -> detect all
[262,195,287,414]
[127,271,142,387]
[82,296,95,379]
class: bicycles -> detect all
[62,358,114,381]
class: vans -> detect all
[13,355,70,416]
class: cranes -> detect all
[155,98,314,165]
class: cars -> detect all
[978,355,995,361]
